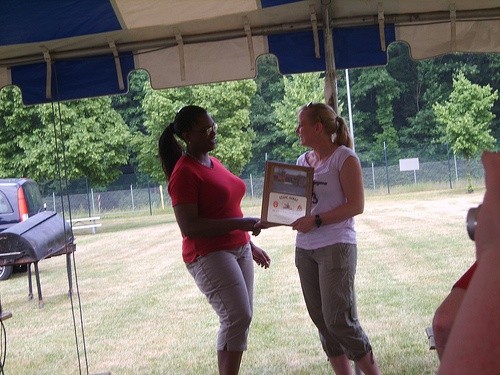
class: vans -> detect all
[0,178,48,281]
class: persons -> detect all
[160,104,273,375]
[253,101,382,375]
[430,148,500,375]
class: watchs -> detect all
[315,214,322,228]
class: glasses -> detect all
[192,123,219,138]
[306,102,318,120]
